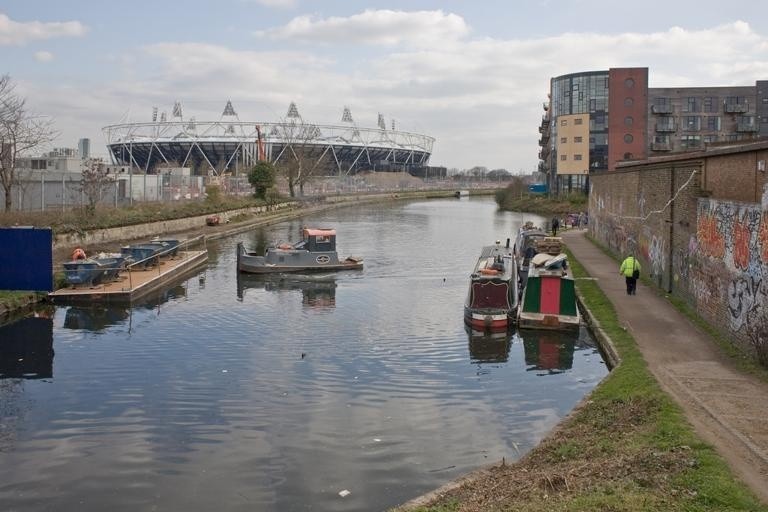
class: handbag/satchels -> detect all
[633,270,639,279]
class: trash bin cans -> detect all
[206,216,219,226]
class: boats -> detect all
[236,227,363,275]
[463,221,580,333]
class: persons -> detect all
[619,253,642,296]
[578,212,585,231]
[551,215,559,237]
